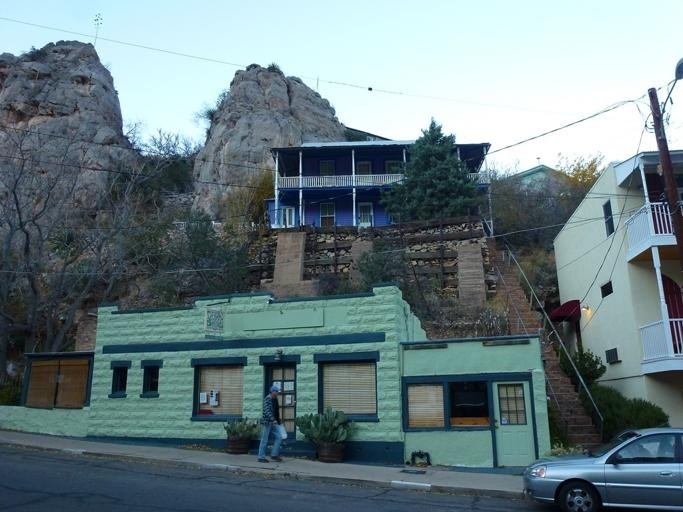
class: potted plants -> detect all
[294,406,359,463]
[222,416,261,454]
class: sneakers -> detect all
[270,456,281,462]
[256,457,269,463]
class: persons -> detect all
[256,386,284,463]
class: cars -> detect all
[522,427,683,512]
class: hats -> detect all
[271,386,281,393]
